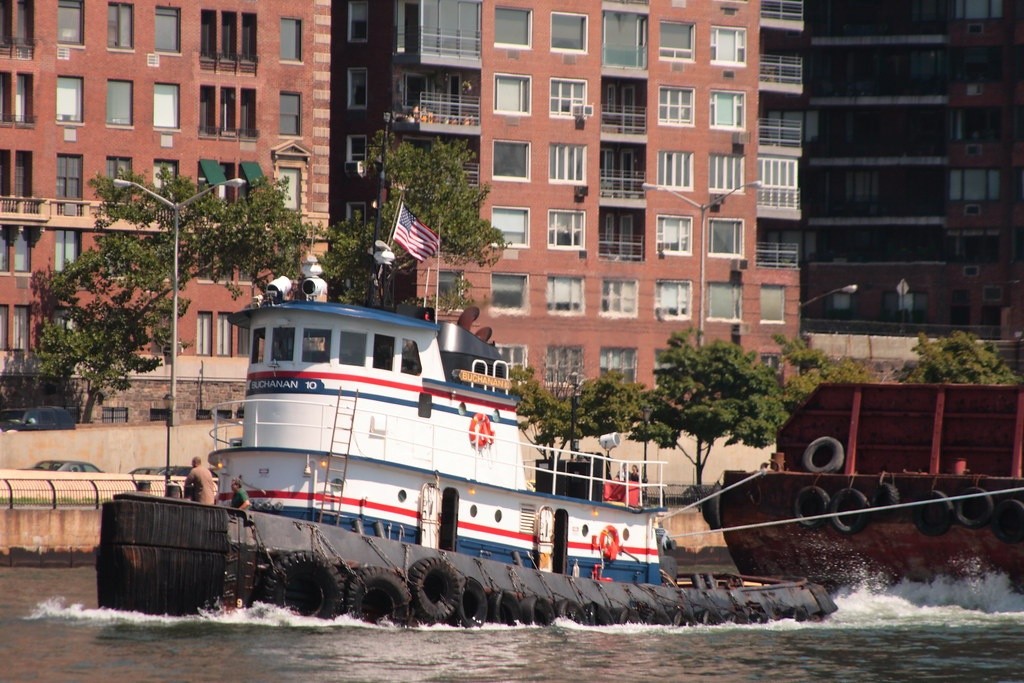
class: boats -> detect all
[94,229,842,626]
[702,378,1024,593]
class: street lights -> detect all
[640,180,772,355]
[798,285,860,379]
[112,176,245,493]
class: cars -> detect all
[129,464,220,477]
[24,460,102,473]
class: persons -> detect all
[185,456,214,506]
[615,462,640,482]
[231,478,250,510]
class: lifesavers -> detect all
[456,573,811,628]
[469,412,491,449]
[909,490,954,537]
[991,499,1024,544]
[346,565,412,628]
[599,525,620,558]
[260,550,344,622]
[829,488,871,536]
[869,482,899,518]
[955,487,996,529]
[791,486,831,529]
[801,436,845,474]
[407,556,461,626]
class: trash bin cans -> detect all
[534,450,606,503]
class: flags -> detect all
[392,201,441,264]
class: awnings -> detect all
[200,159,227,184]
[241,162,265,187]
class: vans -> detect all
[0,405,76,436]
